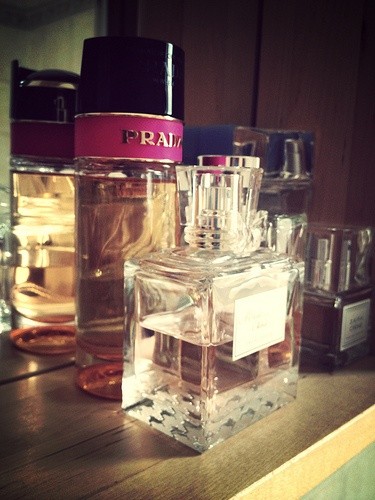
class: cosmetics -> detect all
[286,220,374,367]
[119,152,304,455]
